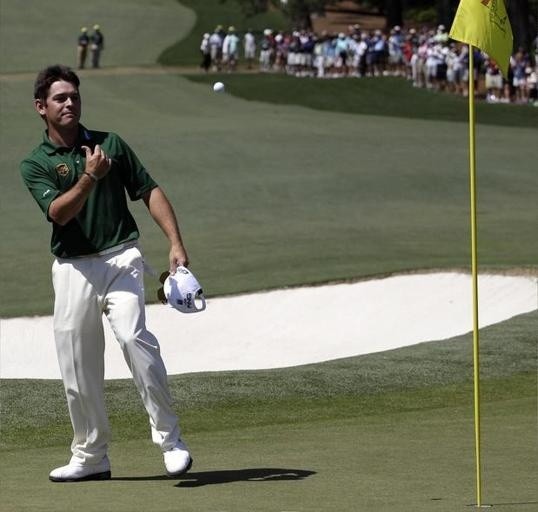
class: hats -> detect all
[264,24,416,44]
[163,266,206,313]
[216,25,236,32]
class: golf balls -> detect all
[213,81,226,93]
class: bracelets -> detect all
[84,170,99,183]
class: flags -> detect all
[449,0,515,81]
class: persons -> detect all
[200,24,239,73]
[259,23,388,78]
[76,27,88,69]
[20,62,194,482]
[243,28,257,69]
[388,25,538,106]
[90,24,105,68]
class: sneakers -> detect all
[49,453,111,482]
[296,70,479,100]
[163,438,193,476]
[487,95,529,105]
